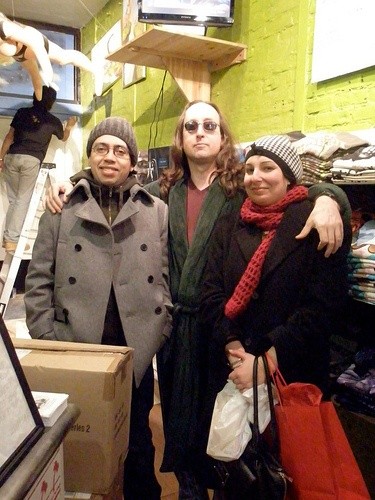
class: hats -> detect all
[87,117,138,166]
[244,135,303,188]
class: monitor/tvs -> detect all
[137,0,234,27]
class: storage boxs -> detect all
[7,337,134,494]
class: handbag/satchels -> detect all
[266,352,372,500]
[224,354,286,500]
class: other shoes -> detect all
[3,239,17,255]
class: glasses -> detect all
[185,121,220,130]
[91,144,130,158]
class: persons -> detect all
[0,85,76,250]
[43,98,353,500]
[25,116,174,500]
[201,135,348,455]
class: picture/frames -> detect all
[0,16,82,104]
